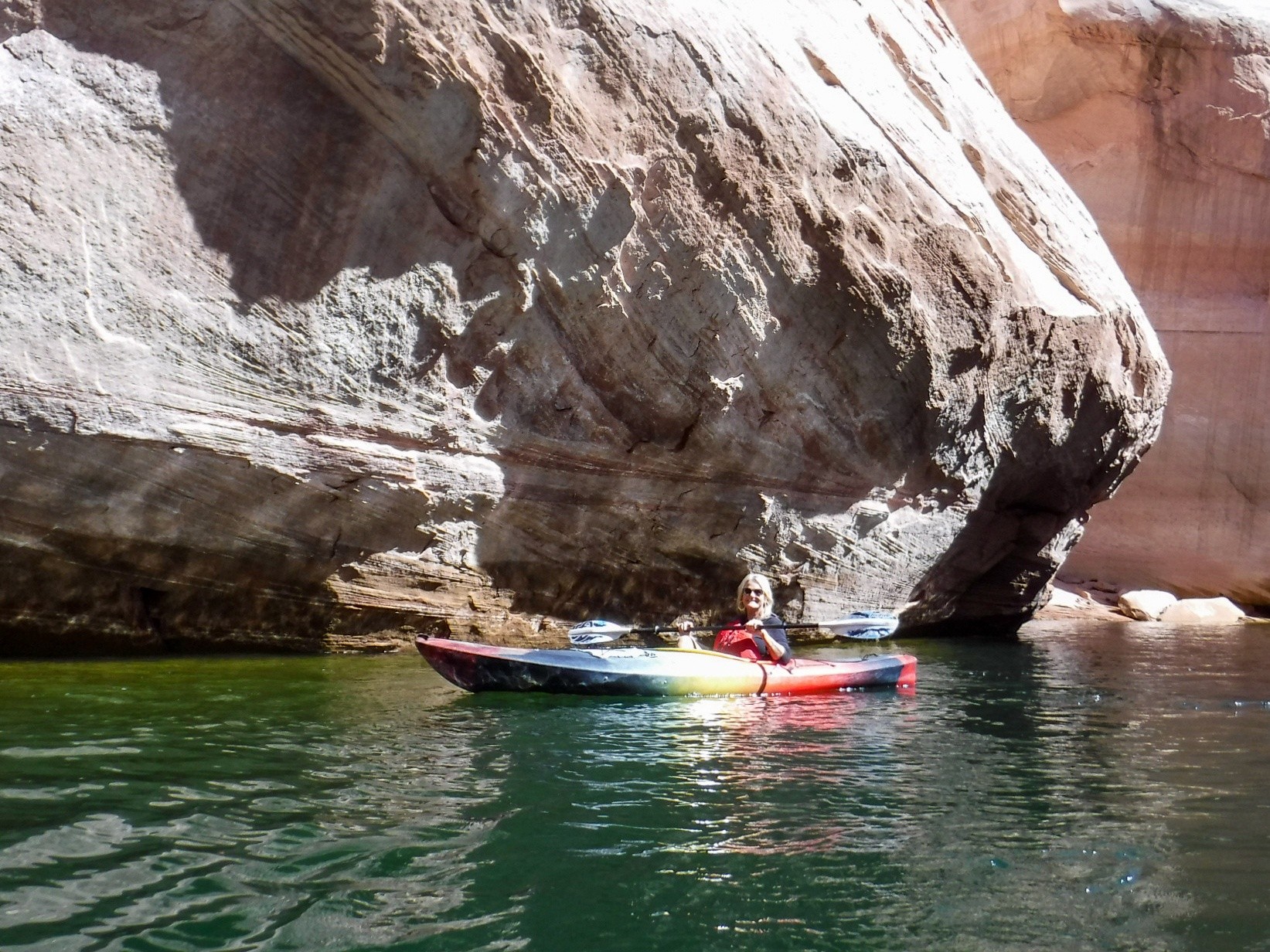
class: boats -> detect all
[415,633,923,695]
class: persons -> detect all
[676,574,792,666]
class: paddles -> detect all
[568,609,904,646]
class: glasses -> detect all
[743,588,763,595]
